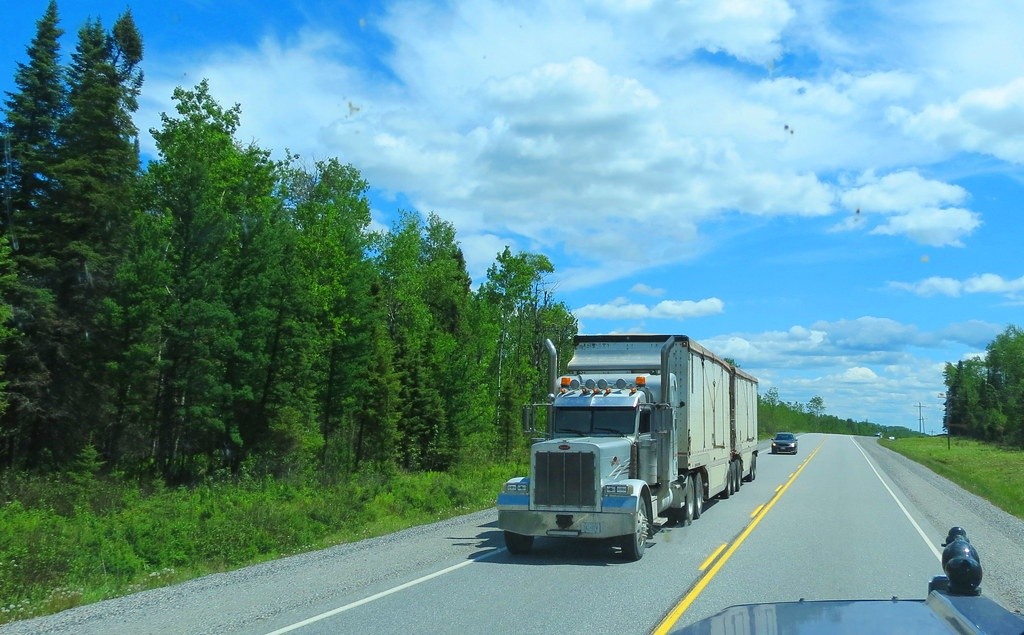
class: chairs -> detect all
[788,435,792,439]
[778,436,782,439]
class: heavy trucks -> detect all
[497,333,761,561]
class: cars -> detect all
[769,432,799,455]
[874,431,884,436]
